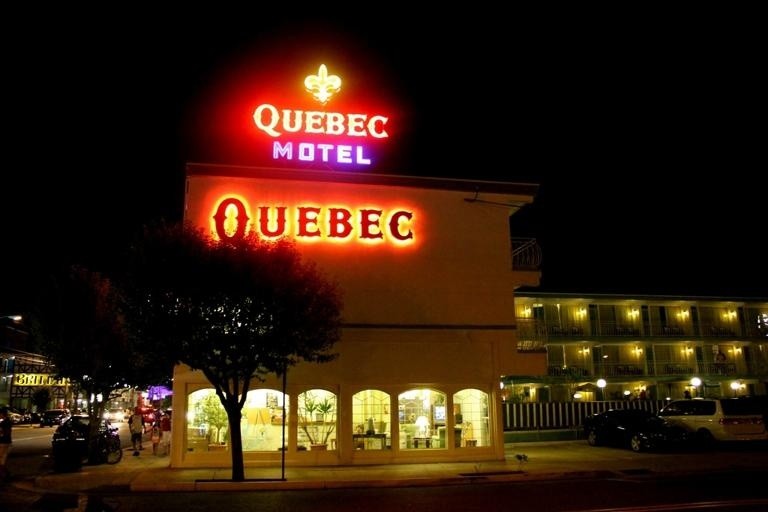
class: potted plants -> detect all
[192,391,228,450]
[305,397,331,451]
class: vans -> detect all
[654,396,767,444]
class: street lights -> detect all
[0,314,23,324]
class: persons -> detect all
[683,389,692,400]
[128,404,171,457]
[0,405,12,465]
[715,348,727,375]
[639,389,647,398]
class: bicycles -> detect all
[93,428,123,464]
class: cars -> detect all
[11,408,32,422]
[585,408,695,452]
[0,412,5,425]
[40,407,72,426]
[74,405,166,427]
[6,408,23,425]
[53,415,108,446]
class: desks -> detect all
[413,436,431,448]
[353,433,387,449]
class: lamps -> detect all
[245,407,272,452]
[415,414,427,437]
